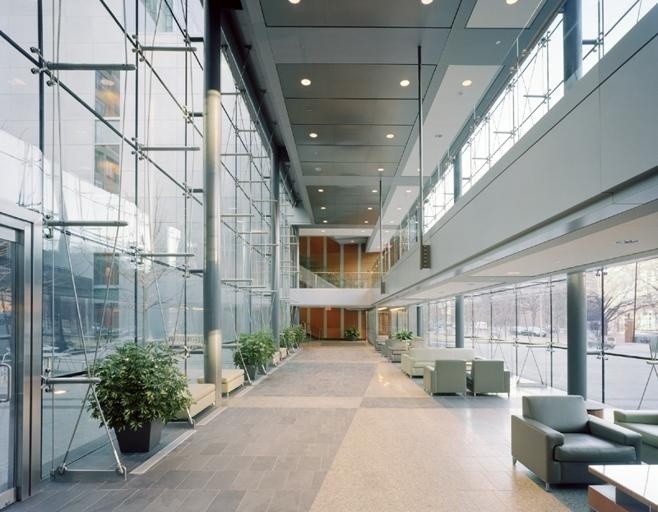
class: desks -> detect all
[585,461,658,511]
[587,401,604,420]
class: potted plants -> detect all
[257,331,278,375]
[234,333,266,382]
[81,340,196,455]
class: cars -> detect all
[428,318,502,338]
[508,324,560,338]
[40,320,136,337]
[634,331,657,343]
[600,334,616,348]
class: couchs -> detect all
[423,359,468,398]
[379,339,390,357]
[432,347,487,373]
[466,359,512,398]
[385,340,407,364]
[399,344,438,380]
[615,410,658,465]
[512,394,646,492]
[409,339,430,349]
[374,334,390,352]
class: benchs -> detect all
[278,345,287,360]
[166,378,218,424]
[217,366,247,395]
[272,350,281,369]
